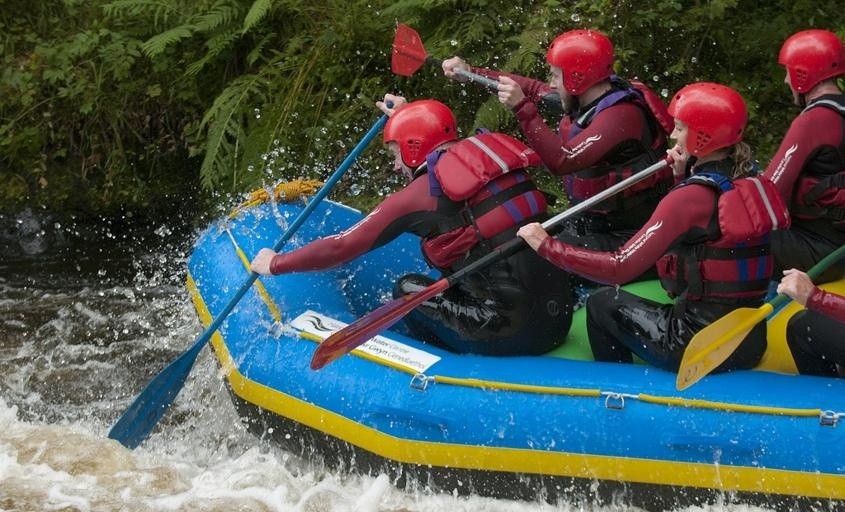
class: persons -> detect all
[249,92,573,355]
[441,28,676,310]
[762,28,845,287]
[776,267,845,378]
[515,81,794,375]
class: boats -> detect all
[188,193,845,507]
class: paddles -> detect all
[107,100,393,451]
[312,148,679,370]
[391,22,499,95]
[676,244,844,391]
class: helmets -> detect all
[778,30,845,94]
[666,83,748,157]
[546,30,614,96]
[382,99,458,169]
